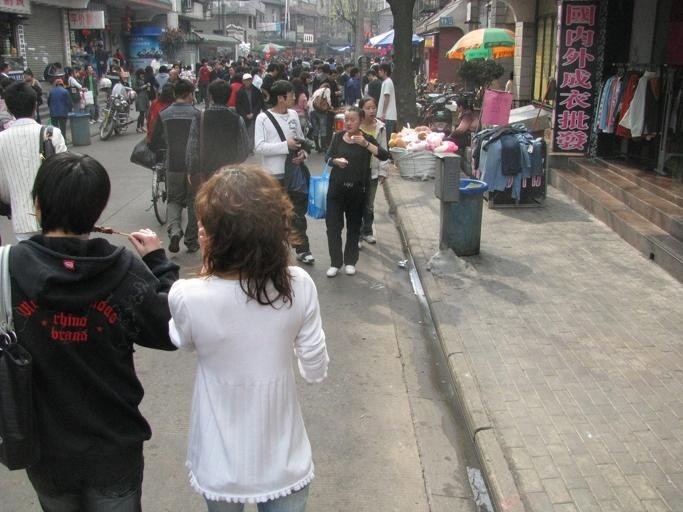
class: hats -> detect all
[242,73,252,80]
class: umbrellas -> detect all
[247,42,288,58]
[366,28,423,54]
[445,27,515,61]
[364,41,393,53]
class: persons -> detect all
[150,77,205,254]
[1,152,181,510]
[147,48,156,58]
[77,40,127,80]
[155,48,164,58]
[137,49,148,58]
[2,53,396,215]
[323,106,392,277]
[168,163,331,510]
[254,79,316,266]
[1,80,69,244]
[182,78,252,255]
[444,97,478,149]
[355,94,391,249]
[504,70,513,93]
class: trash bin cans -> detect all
[439,178,488,257]
[67,112,91,146]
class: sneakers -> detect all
[358,235,363,249]
[168,233,180,253]
[345,265,356,275]
[296,252,314,264]
[89,118,103,124]
[327,267,341,277]
[363,235,376,243]
[187,241,199,252]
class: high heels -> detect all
[136,128,143,133]
[141,128,148,131]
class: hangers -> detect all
[616,62,658,72]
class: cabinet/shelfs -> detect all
[473,133,548,209]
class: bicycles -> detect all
[146,147,169,226]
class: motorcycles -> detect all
[416,74,457,137]
[97,78,138,141]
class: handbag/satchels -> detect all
[187,172,206,201]
[314,96,329,112]
[1,244,39,471]
[84,89,94,104]
[309,158,330,219]
[66,86,80,102]
[285,151,310,197]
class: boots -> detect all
[314,135,319,152]
[320,135,328,151]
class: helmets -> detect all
[98,78,112,91]
